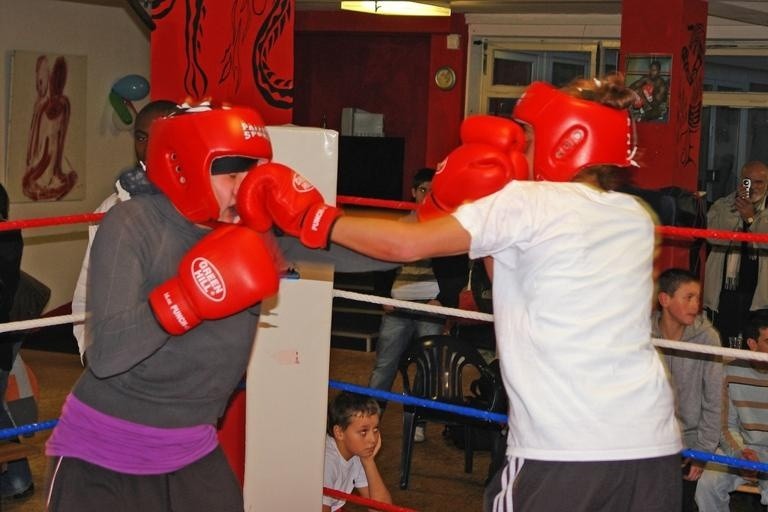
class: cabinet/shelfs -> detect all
[335,133,403,212]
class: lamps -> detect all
[340,1,453,18]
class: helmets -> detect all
[509,79,641,186]
[142,98,274,232]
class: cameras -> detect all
[741,177,754,197]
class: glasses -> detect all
[416,186,432,195]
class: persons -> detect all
[628,62,666,120]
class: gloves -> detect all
[416,112,532,226]
[143,223,282,338]
[234,158,346,255]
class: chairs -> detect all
[395,332,501,492]
